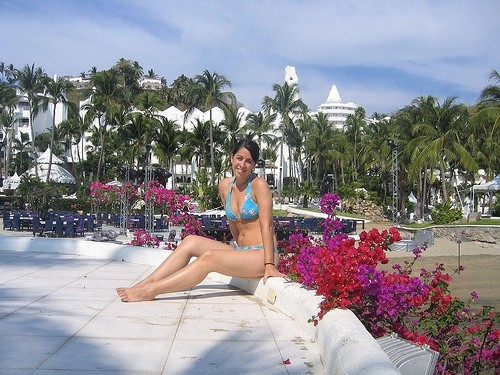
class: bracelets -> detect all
[265,262,275,266]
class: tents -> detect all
[473,173,500,213]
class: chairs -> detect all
[191,213,356,240]
[3,210,170,238]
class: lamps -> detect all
[376,331,439,375]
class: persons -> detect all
[116,139,289,302]
